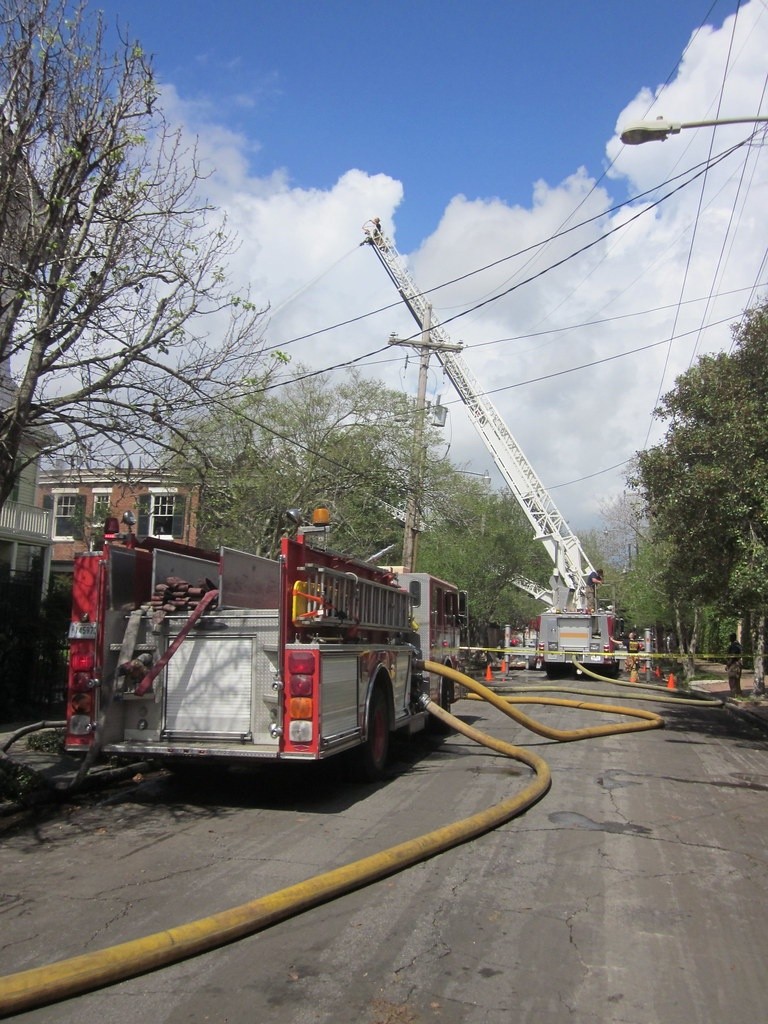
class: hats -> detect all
[729,633,736,636]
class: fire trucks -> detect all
[359,218,624,677]
[63,511,469,780]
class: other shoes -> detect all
[586,610,591,615]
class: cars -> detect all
[508,644,544,670]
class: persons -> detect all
[725,633,742,697]
[586,569,603,615]
[610,632,640,675]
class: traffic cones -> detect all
[502,660,506,672]
[484,665,494,680]
[643,666,646,672]
[655,666,659,676]
[667,673,675,688]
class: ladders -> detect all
[297,562,420,632]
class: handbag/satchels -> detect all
[728,661,741,678]
